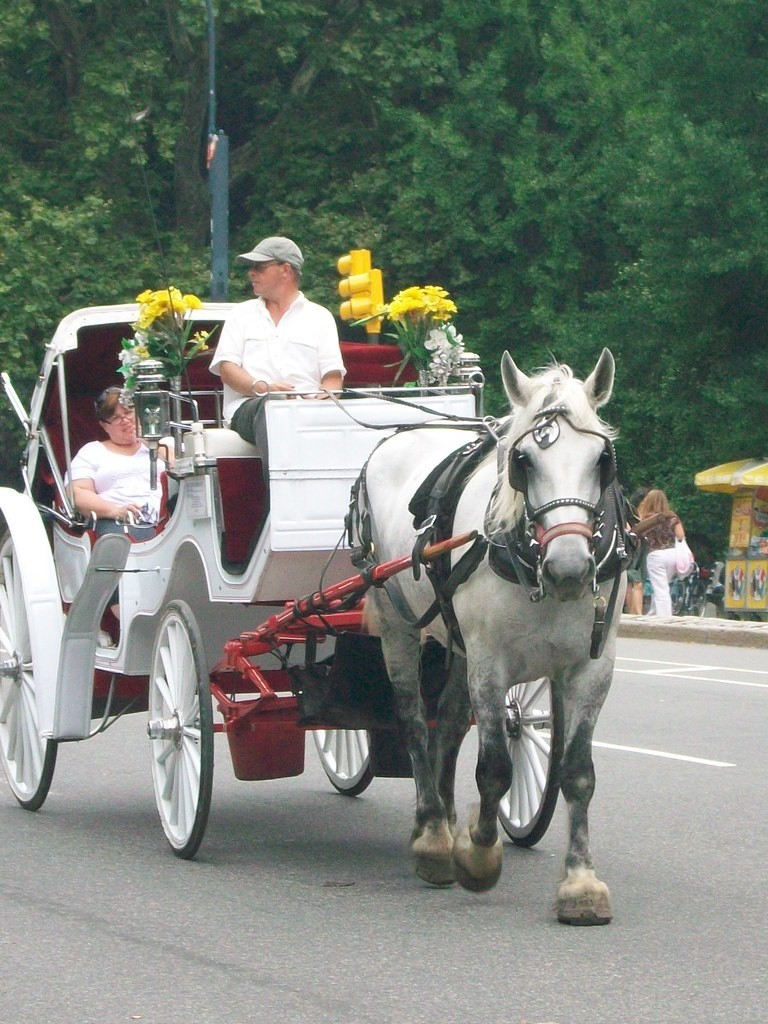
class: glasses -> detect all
[241,262,283,273]
[99,409,136,426]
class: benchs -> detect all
[178,426,264,461]
[50,341,429,646]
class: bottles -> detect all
[183,422,208,458]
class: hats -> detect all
[236,237,304,270]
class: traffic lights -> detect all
[337,248,372,322]
[347,269,384,320]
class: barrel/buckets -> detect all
[217,697,305,782]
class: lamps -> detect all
[129,358,171,444]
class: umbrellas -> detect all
[694,456,768,495]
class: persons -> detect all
[624,487,695,621]
[209,236,347,593]
[62,386,185,623]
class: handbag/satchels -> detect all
[675,535,694,577]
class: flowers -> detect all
[116,286,219,412]
[348,282,466,393]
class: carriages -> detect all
[0,301,681,928]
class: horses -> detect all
[350,345,627,927]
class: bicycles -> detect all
[670,561,707,617]
